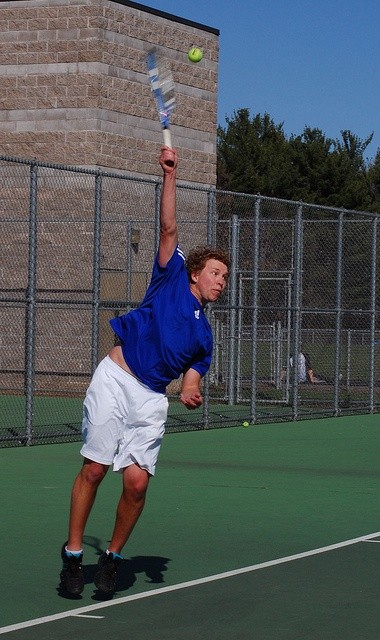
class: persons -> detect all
[280,342,320,386]
[59,146,232,597]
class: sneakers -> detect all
[93,552,122,594]
[61,543,85,597]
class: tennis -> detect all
[188,49,203,63]
[243,422,250,428]
